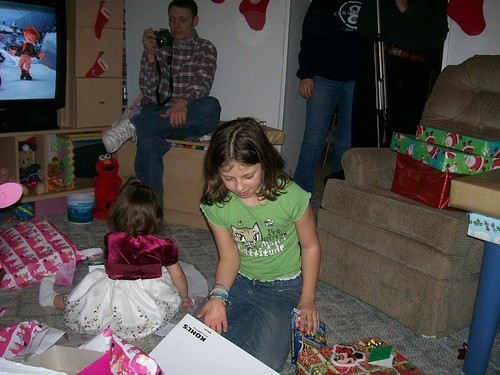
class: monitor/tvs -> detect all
[0,0,66,134]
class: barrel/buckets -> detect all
[66,192,95,224]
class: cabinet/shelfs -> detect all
[0,126,121,203]
[67,0,124,129]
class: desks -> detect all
[449,212,500,374]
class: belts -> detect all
[388,46,419,59]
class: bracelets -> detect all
[207,283,233,307]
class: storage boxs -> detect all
[298,336,424,375]
[390,119,500,219]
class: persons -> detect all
[39,174,209,344]
[294,0,449,191]
[197,116,321,373]
[102,0,222,211]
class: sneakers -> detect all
[102,118,136,153]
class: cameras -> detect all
[154,27,173,47]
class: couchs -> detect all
[314,54,500,338]
